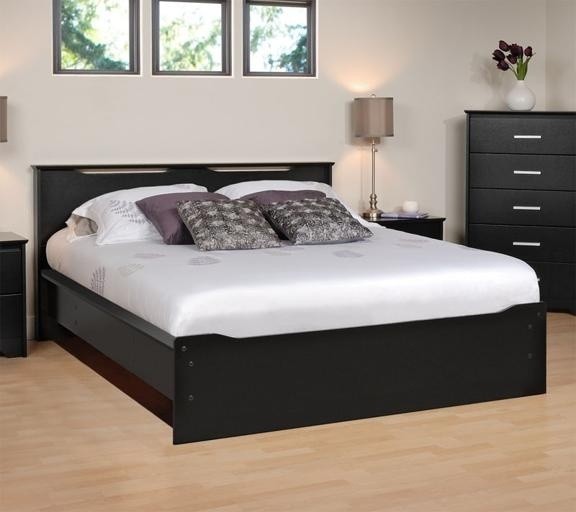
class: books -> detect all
[381,212,430,219]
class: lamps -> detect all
[353,94,395,218]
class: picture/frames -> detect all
[151,0,233,77]
[242,0,317,78]
[52,0,141,76]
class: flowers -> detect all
[492,40,537,79]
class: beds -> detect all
[30,163,547,445]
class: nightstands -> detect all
[368,217,447,241]
[0,233,29,358]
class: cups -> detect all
[403,201,420,214]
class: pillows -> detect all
[223,182,353,243]
[143,192,232,244]
[76,182,212,246]
[178,200,279,247]
[264,197,372,243]
[242,189,337,233]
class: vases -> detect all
[504,81,536,110]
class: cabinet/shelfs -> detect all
[464,109,575,312]
